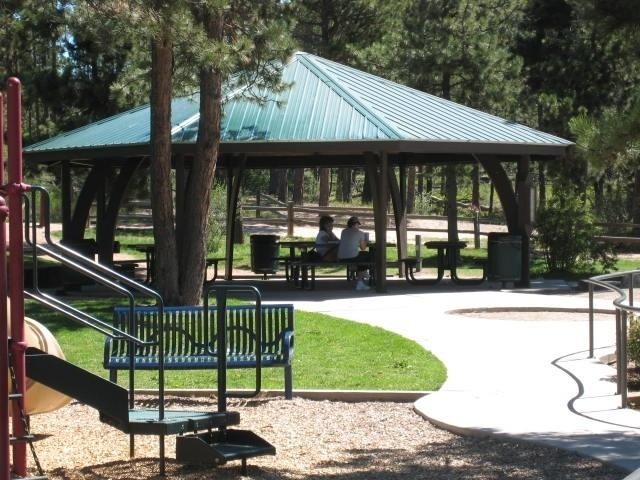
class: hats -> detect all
[347,216,361,225]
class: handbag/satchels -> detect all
[307,250,322,262]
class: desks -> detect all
[424,238,468,286]
[128,243,157,286]
[275,239,396,290]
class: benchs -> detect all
[471,256,490,286]
[204,256,226,285]
[102,303,296,401]
[398,255,424,287]
[272,255,394,289]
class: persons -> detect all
[336,216,375,271]
[315,215,372,291]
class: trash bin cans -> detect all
[487,233,523,288]
[250,235,280,277]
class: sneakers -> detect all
[354,270,371,291]
